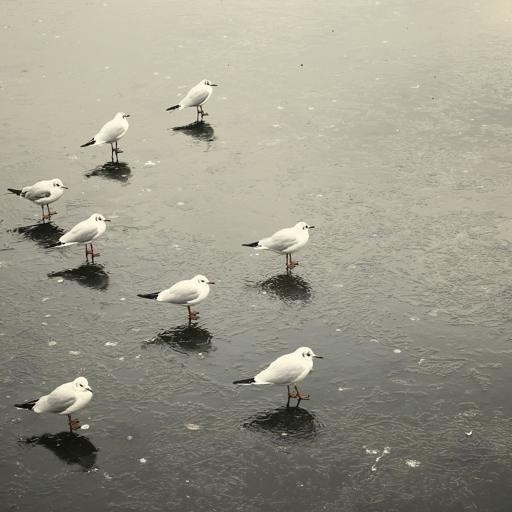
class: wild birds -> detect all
[80,112,131,154]
[242,222,315,269]
[233,346,323,401]
[13,376,93,432]
[165,79,218,116]
[7,178,68,221]
[137,275,216,321]
[44,213,110,257]
